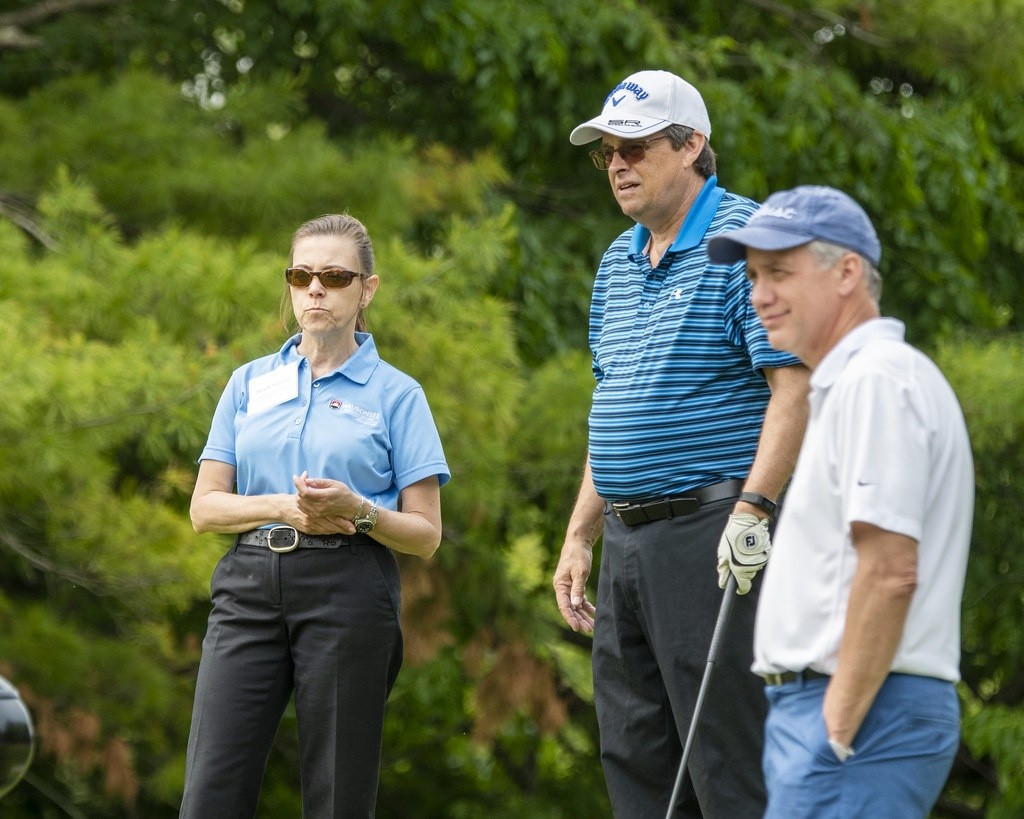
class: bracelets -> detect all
[352,497,365,522]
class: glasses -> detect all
[286,267,365,289]
[588,133,674,170]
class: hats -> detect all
[708,184,882,264]
[569,70,712,145]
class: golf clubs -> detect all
[662,571,740,818]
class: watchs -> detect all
[354,503,380,534]
[737,492,777,519]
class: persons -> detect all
[180,212,452,819]
[552,69,815,819]
[704,184,972,819]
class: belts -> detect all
[605,478,746,527]
[764,667,830,686]
[239,528,390,552]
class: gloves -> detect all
[717,513,772,594]
